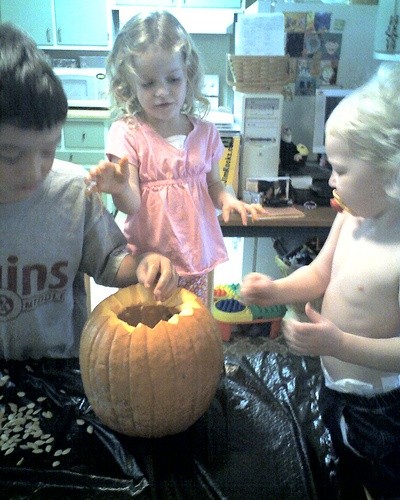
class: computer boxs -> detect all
[233,91,284,199]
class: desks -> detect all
[0,352,340,500]
[208,207,337,312]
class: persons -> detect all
[0,21,178,359]
[83,11,268,307]
[239,91,400,500]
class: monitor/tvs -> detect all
[313,89,354,154]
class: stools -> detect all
[212,283,288,343]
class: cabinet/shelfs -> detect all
[0,0,246,52]
[53,110,117,216]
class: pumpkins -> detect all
[78,283,221,437]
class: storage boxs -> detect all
[225,54,299,93]
